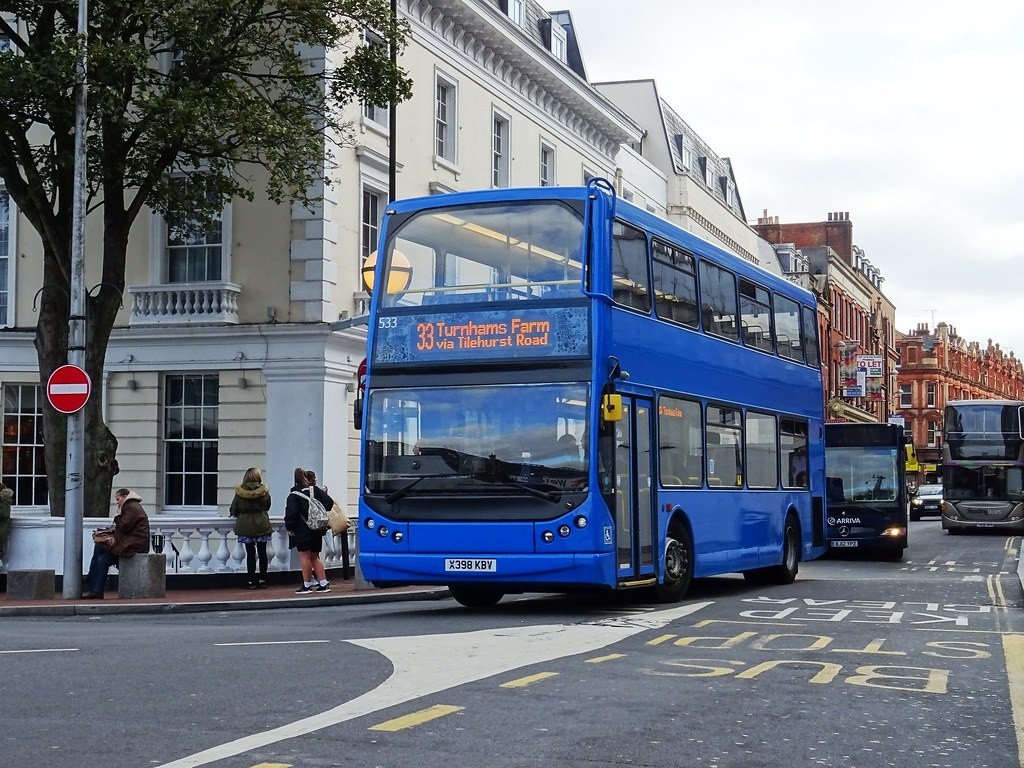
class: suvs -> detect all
[910,484,943,521]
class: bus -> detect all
[825,423,908,562]
[354,177,829,605]
[942,399,1024,535]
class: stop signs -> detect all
[47,363,92,415]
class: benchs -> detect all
[539,277,804,363]
[421,284,520,305]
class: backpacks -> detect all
[291,486,329,531]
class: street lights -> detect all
[935,429,941,461]
[361,250,413,455]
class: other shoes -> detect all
[295,584,313,594]
[248,581,257,589]
[315,582,330,593]
[84,592,104,600]
[82,575,91,583]
[259,582,267,588]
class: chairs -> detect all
[558,434,579,458]
[663,474,722,485]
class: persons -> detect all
[230,467,274,590]
[412,408,810,491]
[295,470,333,586]
[283,468,335,595]
[0,482,13,572]
[905,480,932,504]
[82,488,151,600]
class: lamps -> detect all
[346,383,355,393]
[338,310,349,320]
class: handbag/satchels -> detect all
[326,501,351,535]
